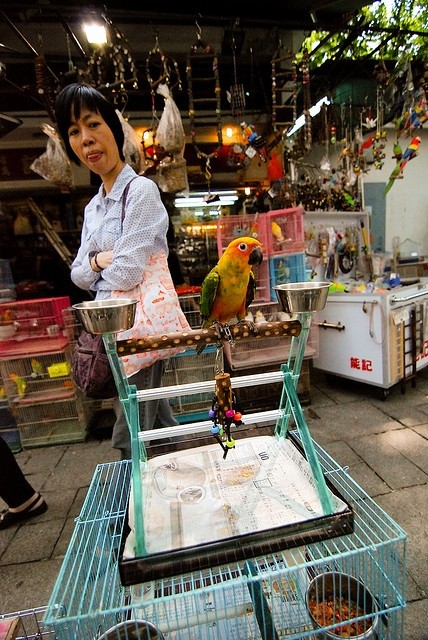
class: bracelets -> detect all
[93,251,104,270]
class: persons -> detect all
[54,85,195,461]
[0,436,49,531]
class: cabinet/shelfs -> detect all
[103,311,354,586]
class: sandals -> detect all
[1,493,48,531]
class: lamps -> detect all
[245,187,251,195]
[64,0,118,56]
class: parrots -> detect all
[382,137,421,198]
[254,310,265,323]
[392,142,402,175]
[245,311,253,323]
[3,309,37,330]
[195,237,262,355]
[249,222,258,239]
[270,221,284,241]
[8,374,26,399]
[30,359,44,378]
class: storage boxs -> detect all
[234,361,311,414]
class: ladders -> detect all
[27,199,94,298]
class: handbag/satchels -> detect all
[69,175,145,399]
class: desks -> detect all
[396,261,428,278]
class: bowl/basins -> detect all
[72,299,140,335]
[97,619,165,640]
[305,571,378,640]
[272,282,333,314]
